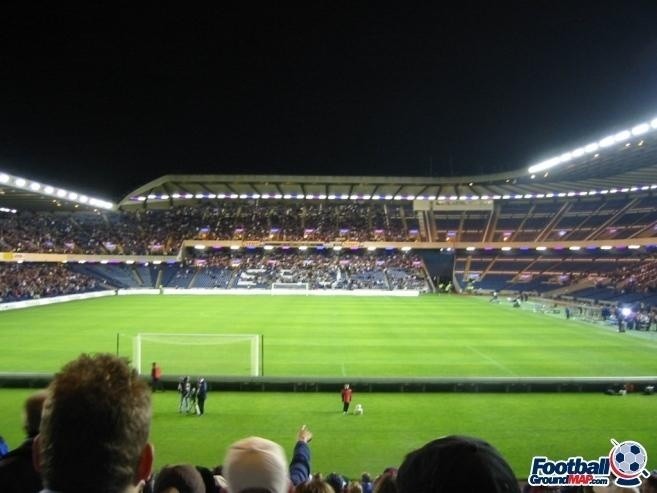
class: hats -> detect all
[396,435,520,491]
[225,436,288,493]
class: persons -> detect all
[0,200,656,333]
[0,351,656,493]
